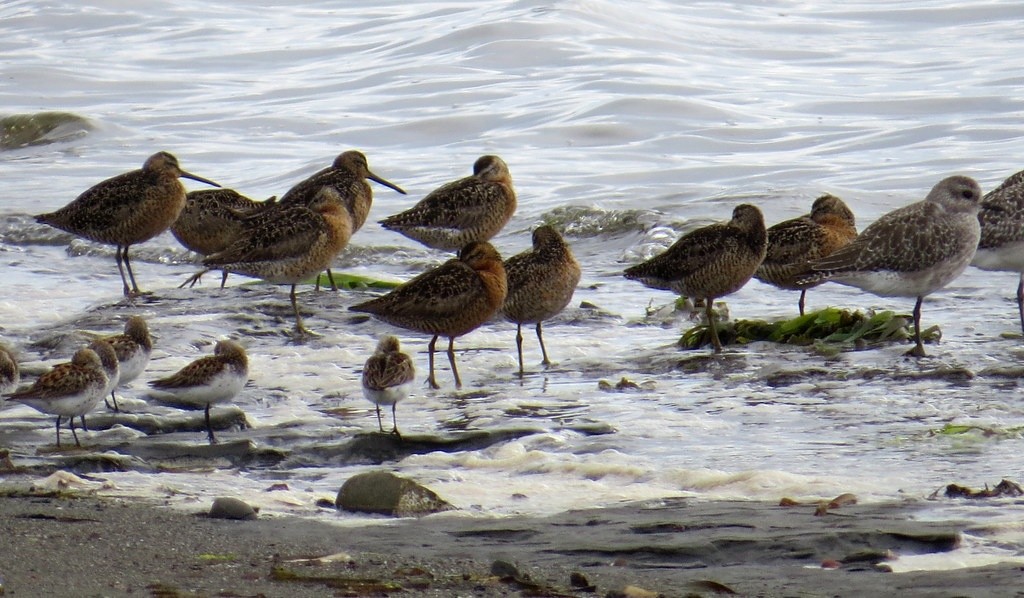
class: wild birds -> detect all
[376,155,519,259]
[624,203,767,354]
[752,194,859,314]
[494,225,582,372]
[347,239,508,391]
[790,175,983,360]
[147,340,250,443]
[167,148,405,346]
[0,316,153,450]
[969,170,1024,334]
[32,149,221,305]
[360,335,416,441]
